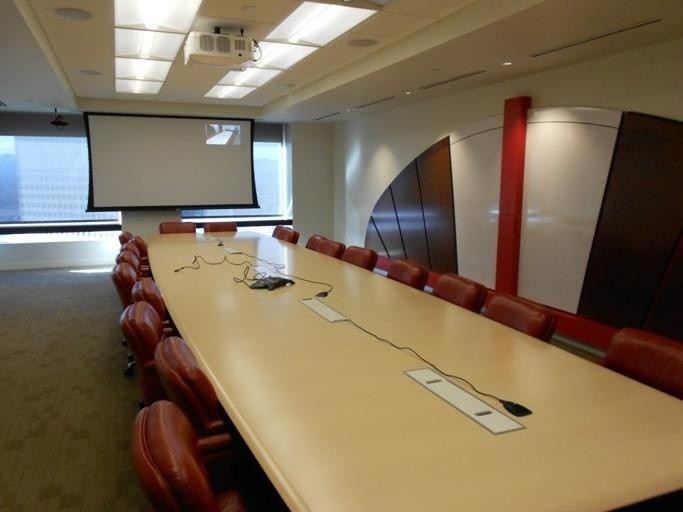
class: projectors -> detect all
[183,31,262,72]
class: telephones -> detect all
[248,275,294,291]
